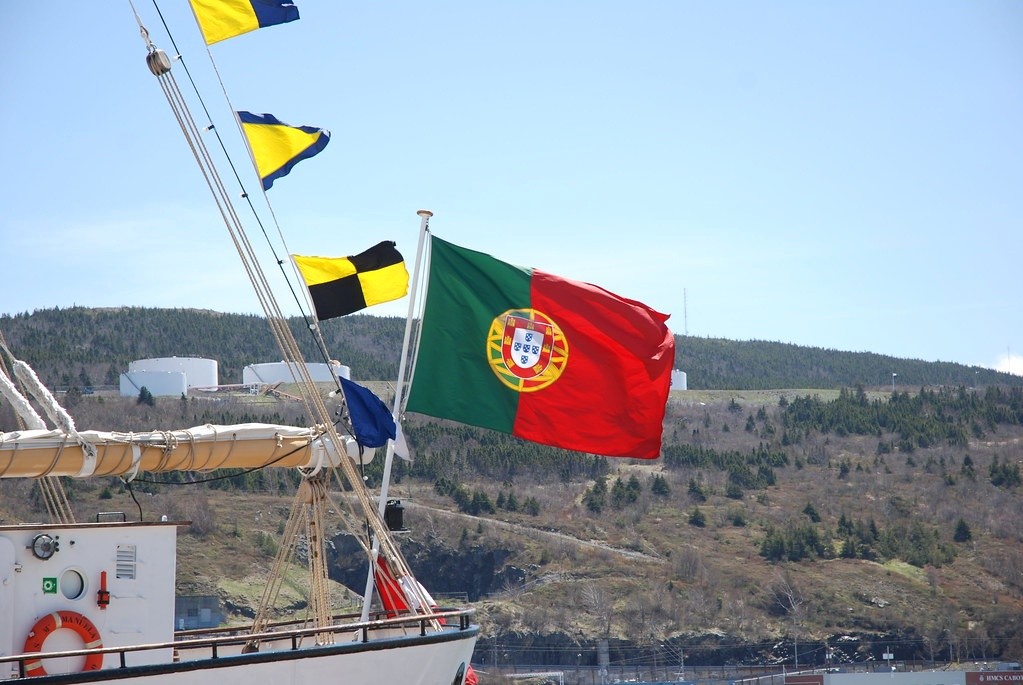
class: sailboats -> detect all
[0,1,481,685]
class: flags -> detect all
[290,240,410,322]
[338,375,411,461]
[401,234,676,460]
[190,0,301,47]
[237,109,331,191]
[371,548,478,685]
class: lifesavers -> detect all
[23,611,104,678]
